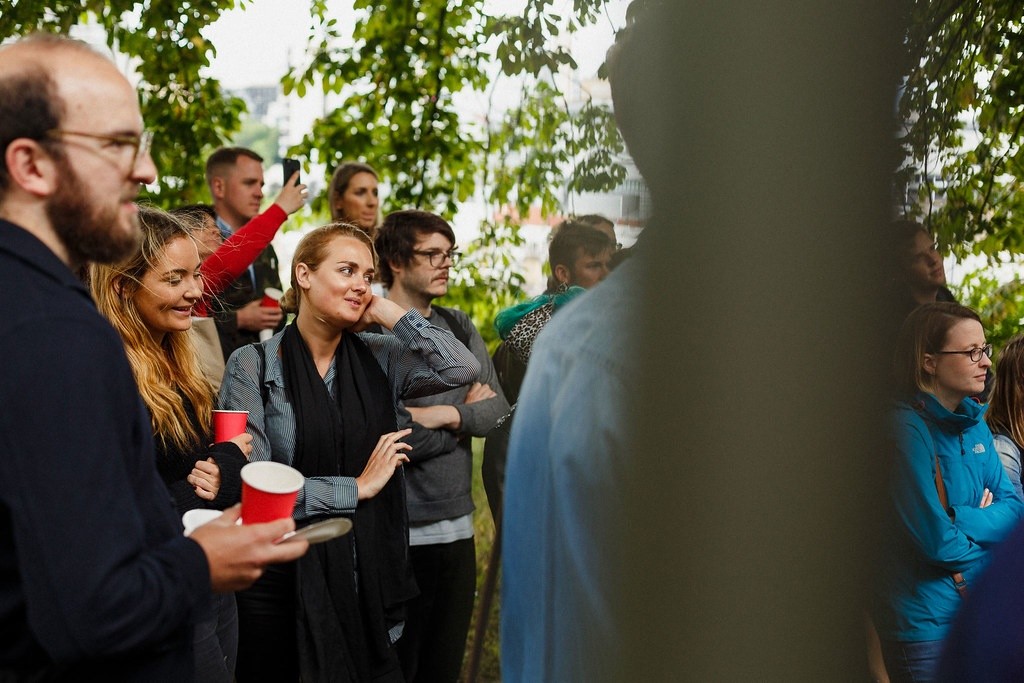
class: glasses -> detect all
[937,345,990,362]
[32,117,154,179]
[413,250,464,267]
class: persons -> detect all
[0,35,311,683]
[481,214,617,540]
[87,147,511,683]
[875,219,1024,683]
[500,0,678,683]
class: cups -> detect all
[261,287,285,308]
[240,460,305,543]
[212,409,249,443]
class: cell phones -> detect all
[282,158,301,188]
